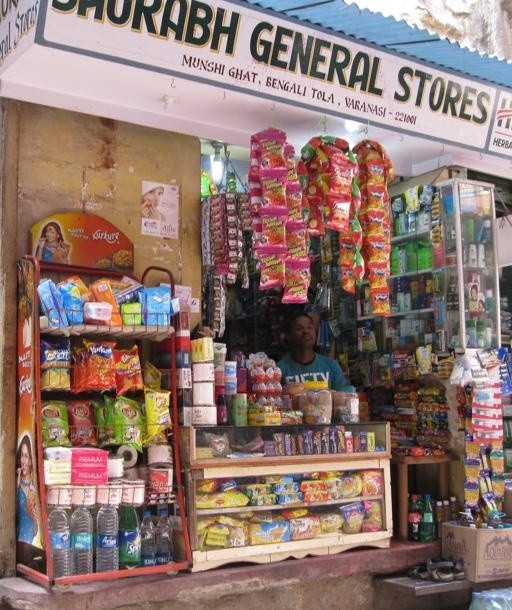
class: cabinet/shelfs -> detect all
[174,418,395,572]
[18,251,190,593]
[233,176,501,364]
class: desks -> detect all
[390,450,463,542]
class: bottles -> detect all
[407,494,462,545]
[216,388,228,424]
[283,380,360,425]
[95,504,119,573]
[155,509,174,567]
[47,508,72,578]
[139,510,157,568]
[69,506,94,576]
[118,502,141,569]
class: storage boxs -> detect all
[440,518,512,585]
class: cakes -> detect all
[95,257,112,271]
[112,249,132,270]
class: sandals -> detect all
[405,556,466,582]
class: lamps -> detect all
[211,140,226,183]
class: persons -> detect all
[142,180,166,222]
[478,299,485,312]
[34,222,72,265]
[189,274,251,361]
[468,284,478,313]
[275,311,348,390]
[14,432,43,551]
